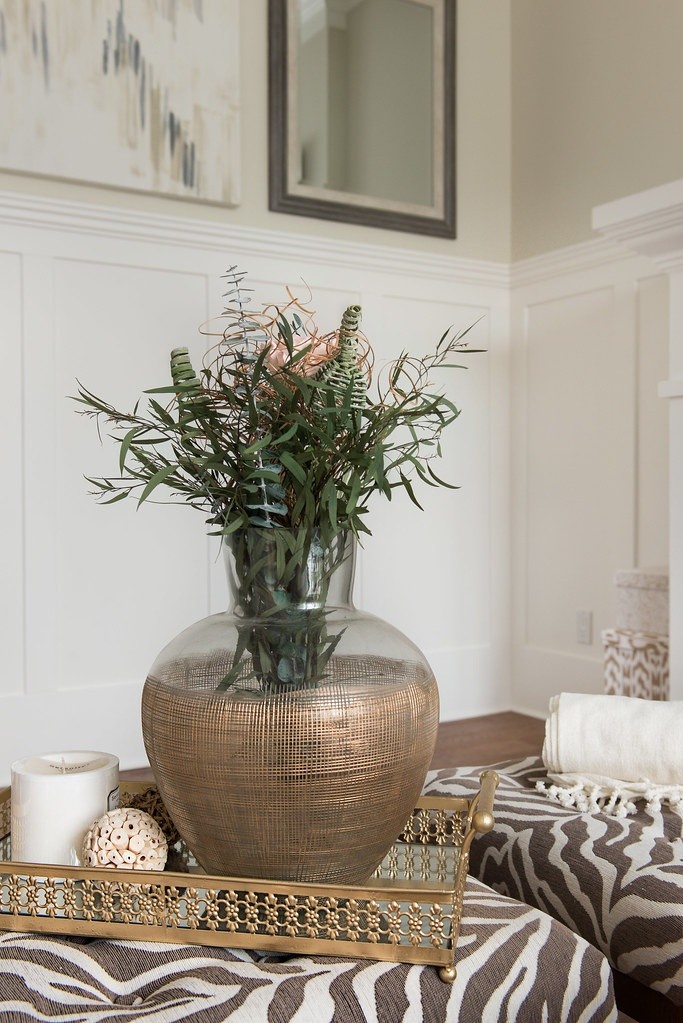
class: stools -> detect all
[0,840,618,1023]
[418,753,683,1023]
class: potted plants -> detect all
[62,262,489,885]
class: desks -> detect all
[0,709,547,834]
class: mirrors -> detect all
[267,0,459,242]
